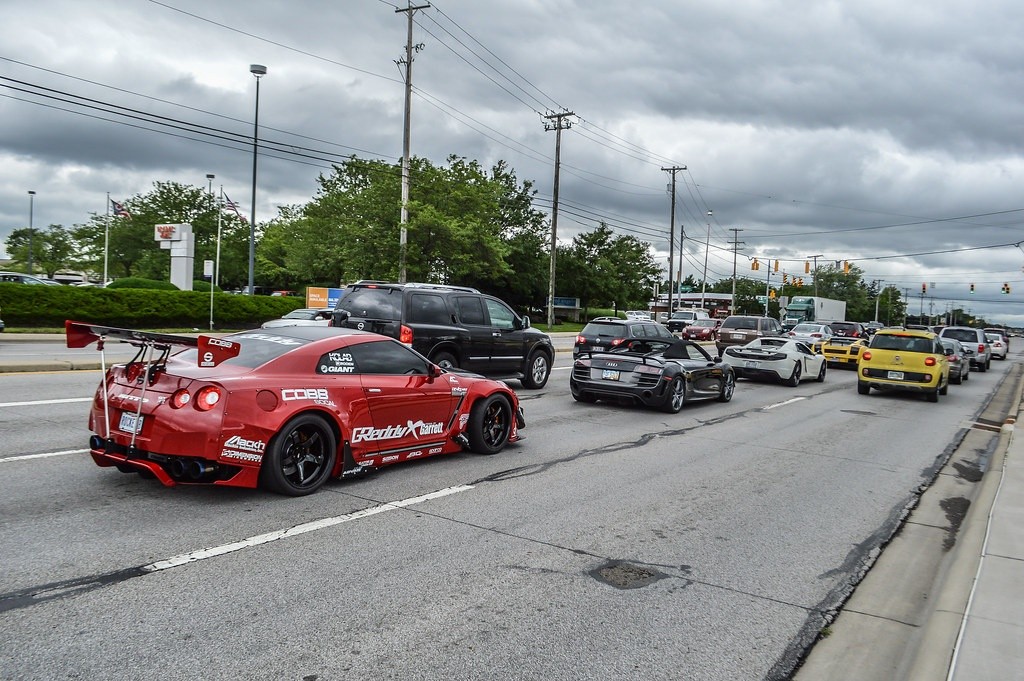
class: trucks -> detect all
[780,295,847,330]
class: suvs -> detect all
[856,328,955,402]
[982,328,1011,353]
[331,280,556,390]
[665,309,709,333]
[715,314,786,357]
[939,324,994,372]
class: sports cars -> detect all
[569,338,737,414]
[62,316,526,497]
[720,335,827,387]
[812,335,870,366]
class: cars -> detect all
[786,323,833,343]
[260,307,335,329]
[984,333,1008,360]
[0,269,107,289]
[223,286,304,297]
[624,309,674,323]
[828,320,949,340]
[571,316,680,362]
[681,318,724,342]
[932,337,974,384]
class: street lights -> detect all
[27,189,37,275]
[205,174,215,259]
[248,63,268,295]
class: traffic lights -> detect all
[970,285,975,294]
[797,278,804,287]
[1001,283,1009,294]
[921,283,927,294]
[783,274,788,283]
[792,278,796,286]
[755,263,760,270]
